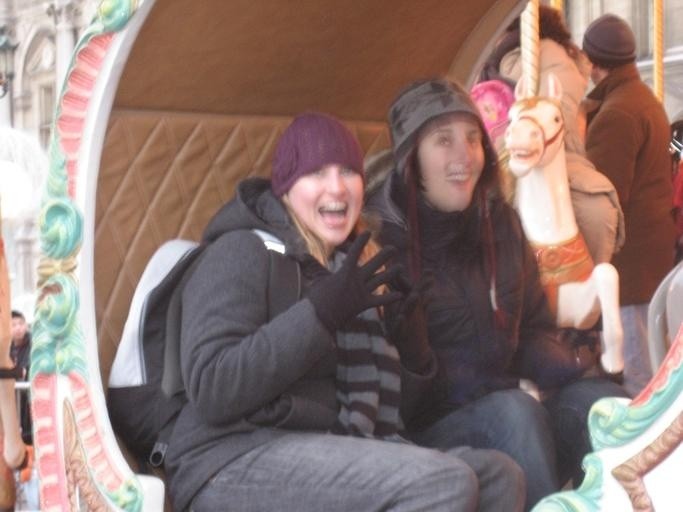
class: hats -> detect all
[271,113,365,199]
[583,15,636,63]
[387,79,480,151]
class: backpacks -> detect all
[108,229,286,467]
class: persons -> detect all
[359,77,634,511]
[9,311,33,445]
[162,115,528,512]
[584,12,683,400]
[478,5,624,265]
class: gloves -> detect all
[300,231,433,367]
